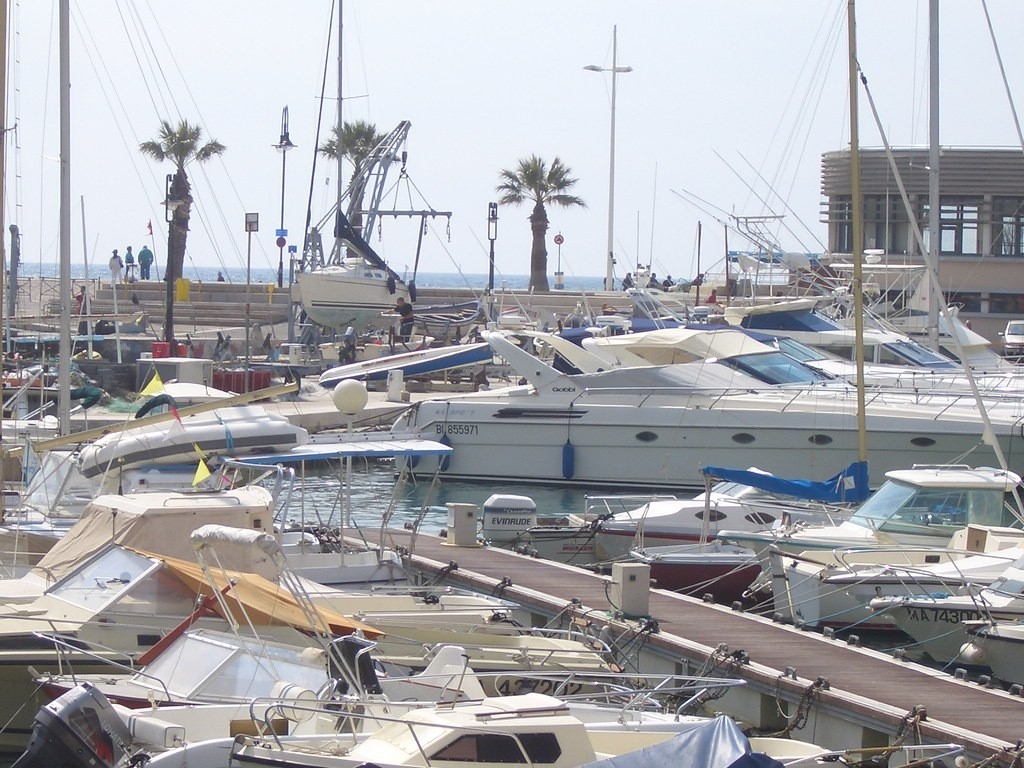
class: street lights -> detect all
[270,104,298,287]
[582,24,635,290]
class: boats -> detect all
[1,0,1024,768]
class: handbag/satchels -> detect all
[119,256,123,268]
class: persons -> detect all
[125,246,135,279]
[707,288,718,303]
[76,286,92,314]
[604,273,704,291]
[138,246,153,280]
[217,271,224,281]
[109,250,123,289]
[384,297,414,342]
